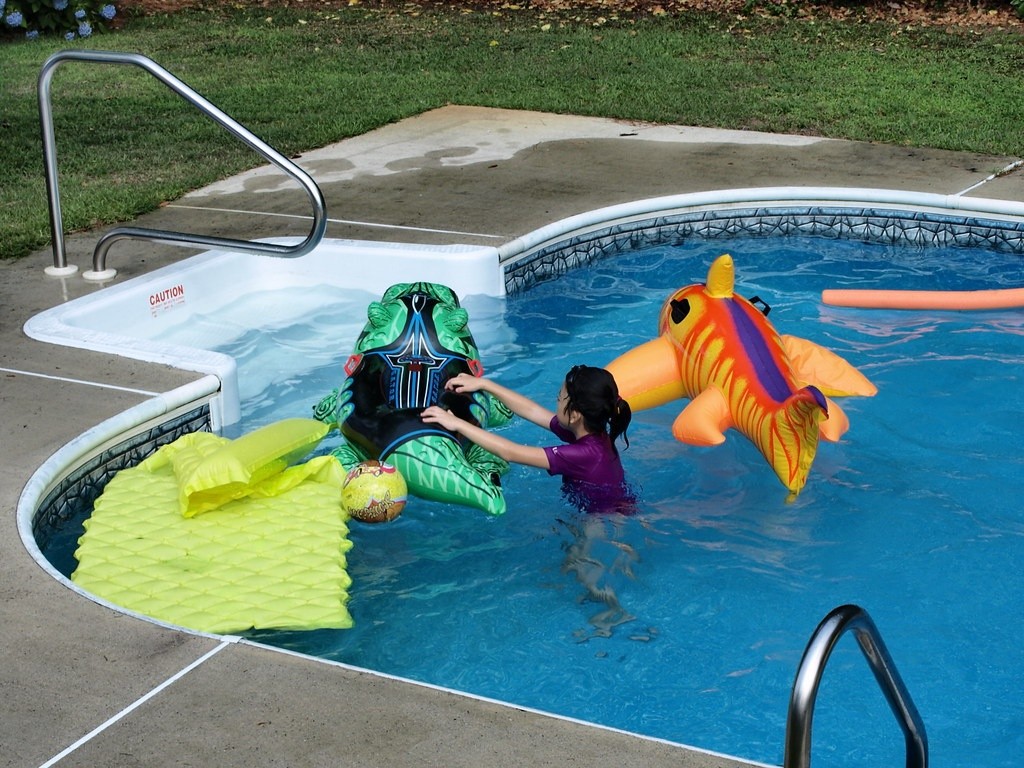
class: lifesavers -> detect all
[821,287,1024,312]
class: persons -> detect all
[420,364,637,515]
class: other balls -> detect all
[341,459,408,524]
